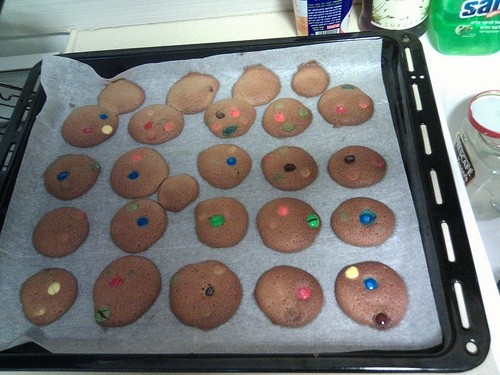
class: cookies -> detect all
[21,255,409,330]
[33,196,397,257]
[62,60,374,148]
[44,144,387,212]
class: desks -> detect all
[64,10,500,375]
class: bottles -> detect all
[293,0,353,38]
[358,1,431,40]
[426,0,500,56]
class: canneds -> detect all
[427,0,500,56]
